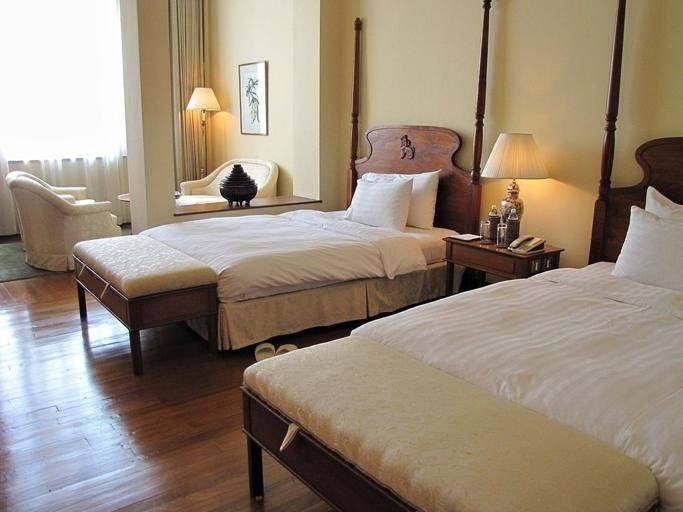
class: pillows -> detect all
[610,206,682,291]
[362,168,443,229]
[645,186,682,219]
[343,177,413,232]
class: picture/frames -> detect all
[238,60,268,136]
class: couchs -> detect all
[6,170,122,272]
[180,158,279,206]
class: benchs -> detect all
[240,333,659,512]
[73,234,218,377]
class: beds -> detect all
[139,0,491,351]
[351,0,682,510]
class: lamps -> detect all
[187,87,221,178]
[481,132,550,242]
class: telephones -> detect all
[507,235,546,255]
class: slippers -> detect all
[255,342,275,361]
[276,344,298,356]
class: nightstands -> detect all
[443,235,566,297]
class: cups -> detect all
[479,221,492,246]
[496,223,508,249]
